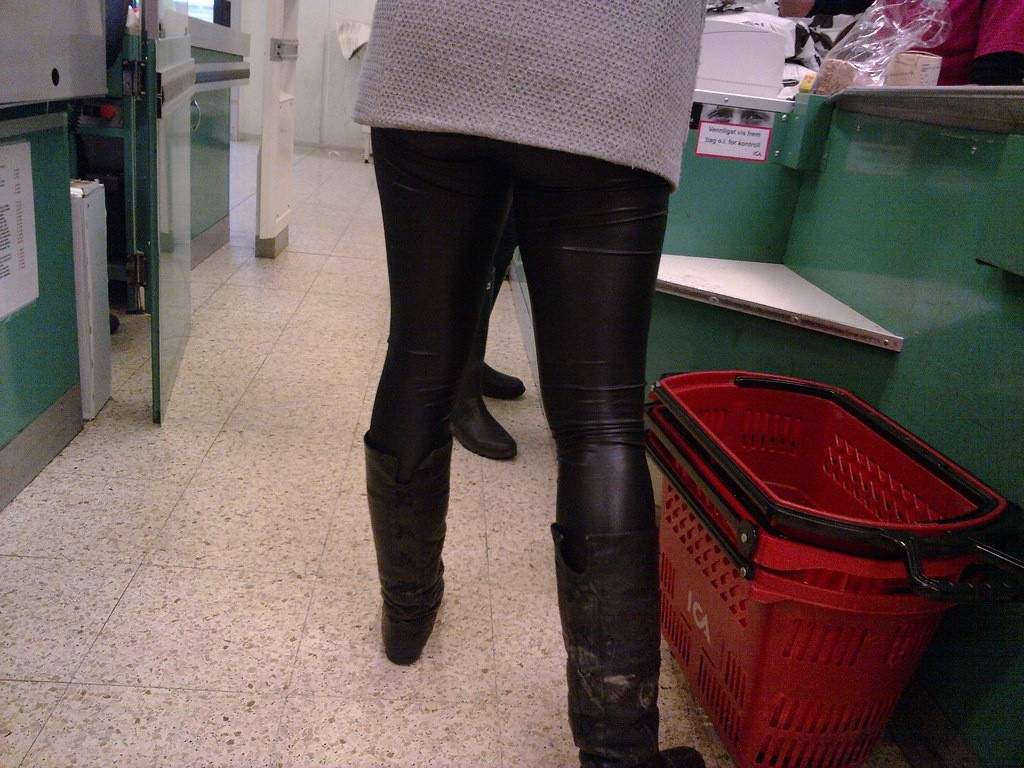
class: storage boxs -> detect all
[636,368,1006,768]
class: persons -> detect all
[345,0,711,767]
[449,201,526,463]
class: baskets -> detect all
[644,362,1009,768]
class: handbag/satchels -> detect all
[809,0,954,95]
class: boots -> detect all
[358,428,450,665]
[546,520,704,768]
[482,263,526,400]
[448,266,518,460]
